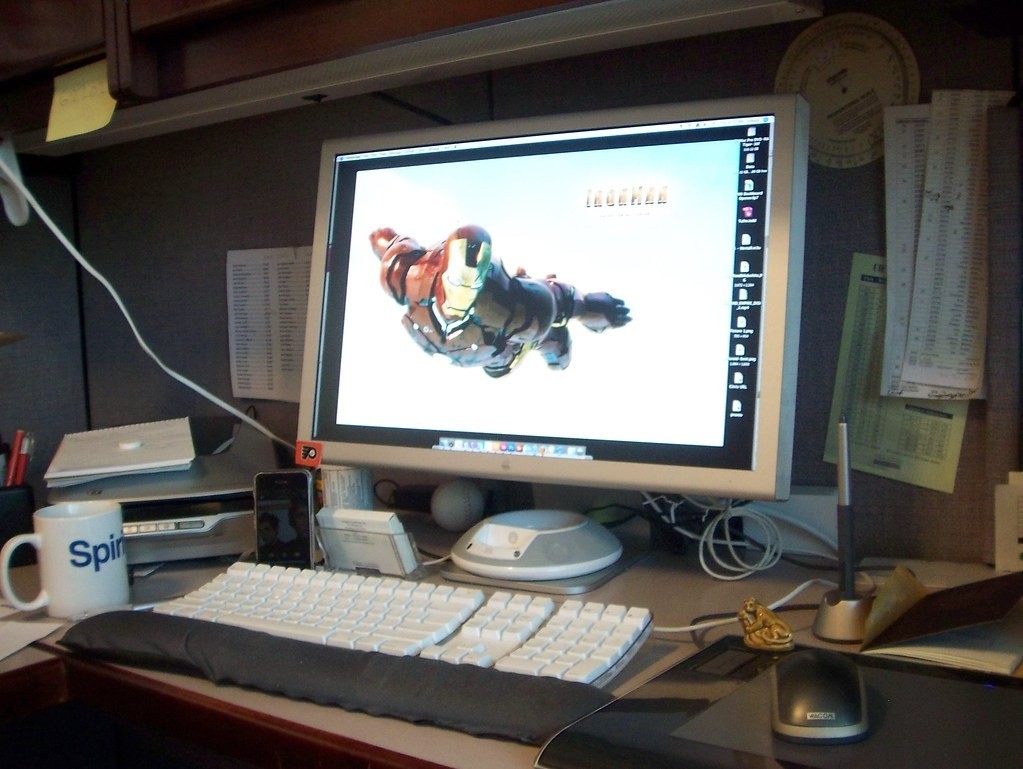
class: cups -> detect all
[0,499,132,621]
[0,483,35,567]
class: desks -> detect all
[0,484,1023,769]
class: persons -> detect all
[371,225,632,378]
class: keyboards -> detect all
[154,561,655,690]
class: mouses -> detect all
[772,649,869,745]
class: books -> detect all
[859,570,1023,676]
[44,417,196,487]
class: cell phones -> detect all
[253,470,316,572]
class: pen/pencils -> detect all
[5,428,36,486]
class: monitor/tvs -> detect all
[295,92,811,592]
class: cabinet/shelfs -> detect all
[0,0,822,162]
